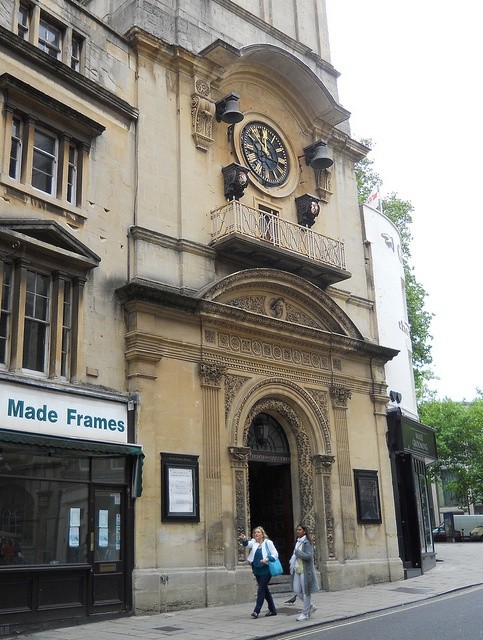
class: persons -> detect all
[239,526,283,618]
[288,524,320,622]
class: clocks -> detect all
[234,109,301,199]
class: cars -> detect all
[427,527,461,543]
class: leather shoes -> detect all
[251,612,258,617]
[265,611,276,616]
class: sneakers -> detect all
[296,611,309,621]
[310,606,316,612]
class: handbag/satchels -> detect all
[265,541,283,576]
